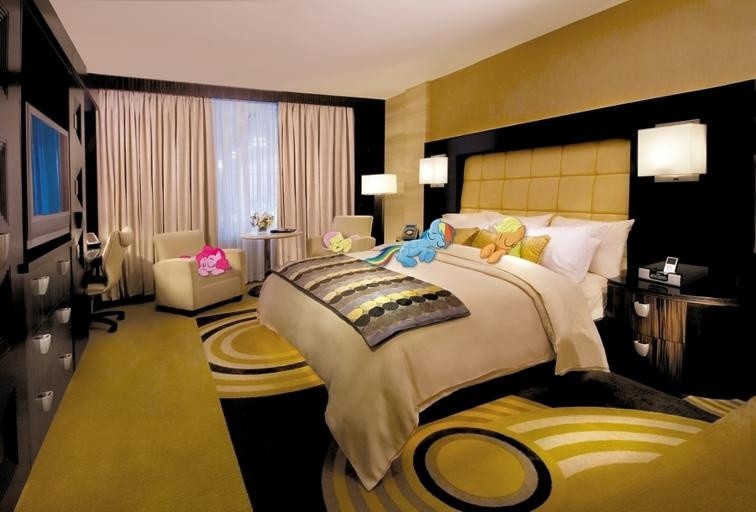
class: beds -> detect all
[255,239,632,492]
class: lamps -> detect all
[417,152,449,190]
[636,118,709,185]
[360,171,399,246]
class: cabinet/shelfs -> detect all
[7,241,75,470]
[68,84,90,350]
[2,1,23,266]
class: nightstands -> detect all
[605,274,744,399]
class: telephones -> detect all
[403,225,420,241]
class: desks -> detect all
[239,230,304,297]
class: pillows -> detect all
[438,210,638,286]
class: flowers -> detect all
[244,206,275,238]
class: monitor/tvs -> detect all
[25,101,70,248]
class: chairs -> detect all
[84,225,135,333]
[305,213,377,259]
[150,228,247,318]
